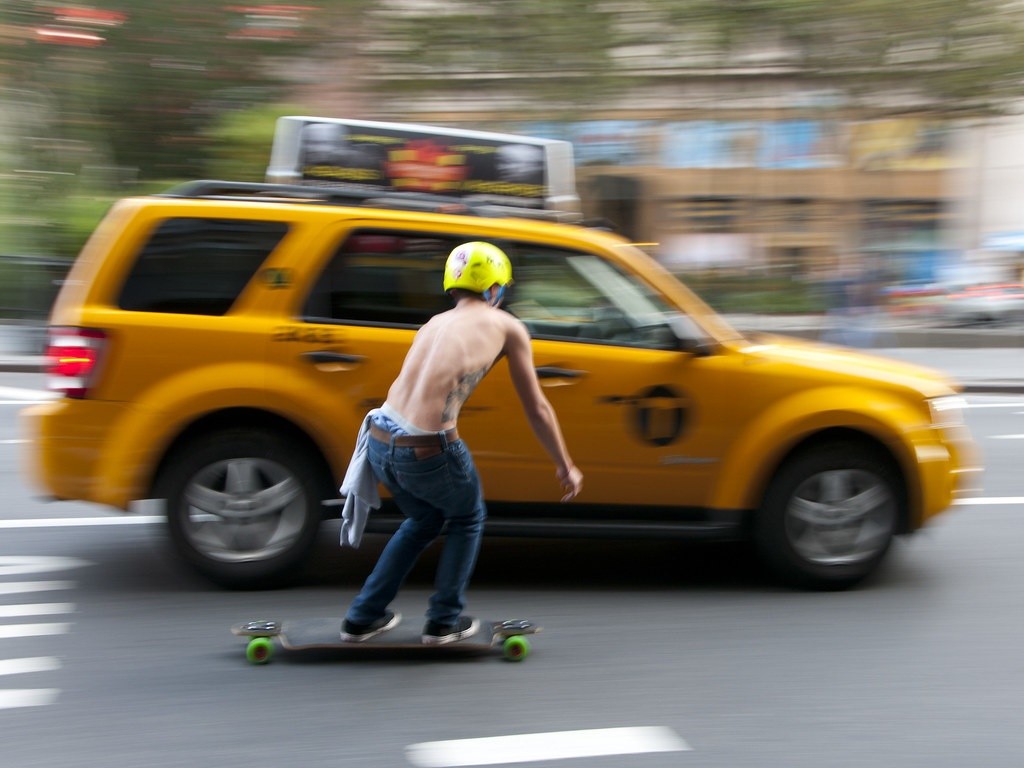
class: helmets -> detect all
[442,242,513,294]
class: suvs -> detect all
[39,122,975,582]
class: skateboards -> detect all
[229,618,546,665]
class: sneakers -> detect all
[422,614,480,645]
[341,609,402,641]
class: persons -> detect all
[340,241,584,644]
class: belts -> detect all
[369,421,459,448]
